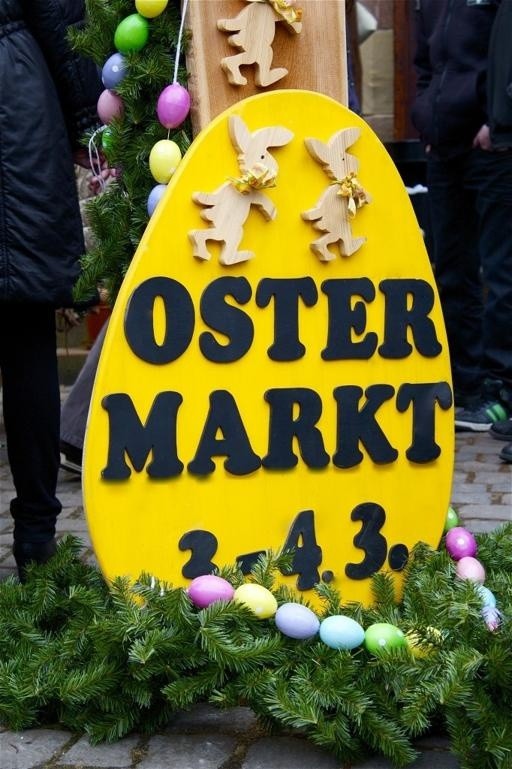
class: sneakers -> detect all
[454,398,512,432]
[58,438,82,475]
[11,536,61,590]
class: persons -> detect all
[0,0,86,585]
[409,0,512,433]
[491,417,511,462]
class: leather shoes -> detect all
[489,418,512,441]
[498,442,511,464]
